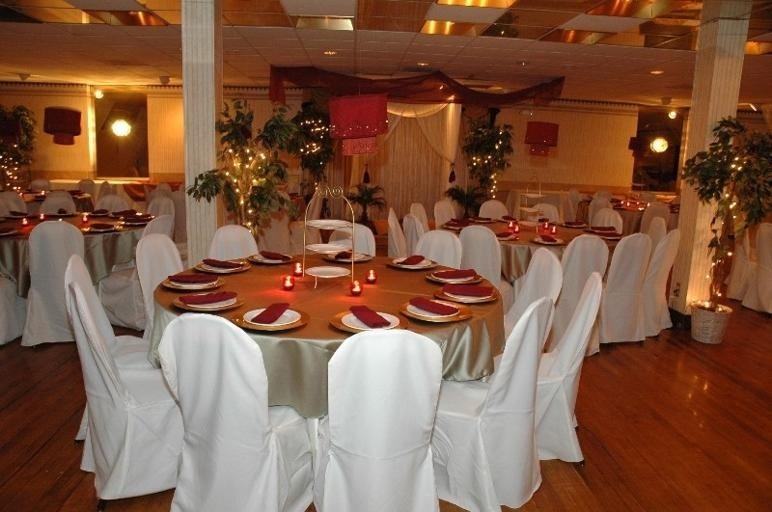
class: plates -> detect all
[0,185,85,202]
[340,311,399,330]
[392,255,431,268]
[431,286,498,306]
[338,250,374,265]
[386,254,436,270]
[169,295,247,313]
[252,253,293,264]
[232,308,311,330]
[441,214,624,243]
[328,308,410,335]
[178,294,236,309]
[246,250,295,266]
[162,276,226,293]
[306,242,349,257]
[424,269,486,285]
[304,264,351,280]
[1,208,155,237]
[192,260,251,277]
[244,307,299,325]
[586,191,683,211]
[443,287,495,301]
[169,277,220,289]
[430,271,474,282]
[406,302,460,318]
[200,262,243,272]
[400,299,473,325]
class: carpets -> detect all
[386,200,512,296]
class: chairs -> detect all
[310,328,444,512]
[541,234,610,361]
[155,312,314,512]
[0,255,28,346]
[62,254,151,443]
[207,223,260,263]
[428,296,556,512]
[21,221,85,346]
[644,228,680,338]
[489,271,604,463]
[597,233,652,343]
[63,280,184,512]
[502,247,564,344]
[327,220,376,257]
[1,174,187,245]
[478,198,510,222]
[95,214,183,338]
[517,193,671,240]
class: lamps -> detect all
[629,128,682,179]
[328,0,388,141]
[43,107,81,146]
[524,121,559,157]
[341,137,379,157]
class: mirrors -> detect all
[86,84,152,182]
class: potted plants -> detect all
[679,115,772,345]
